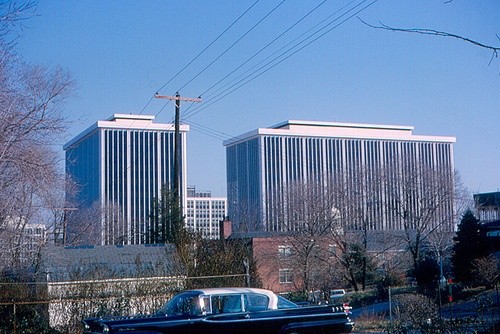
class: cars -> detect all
[82,287,353,334]
